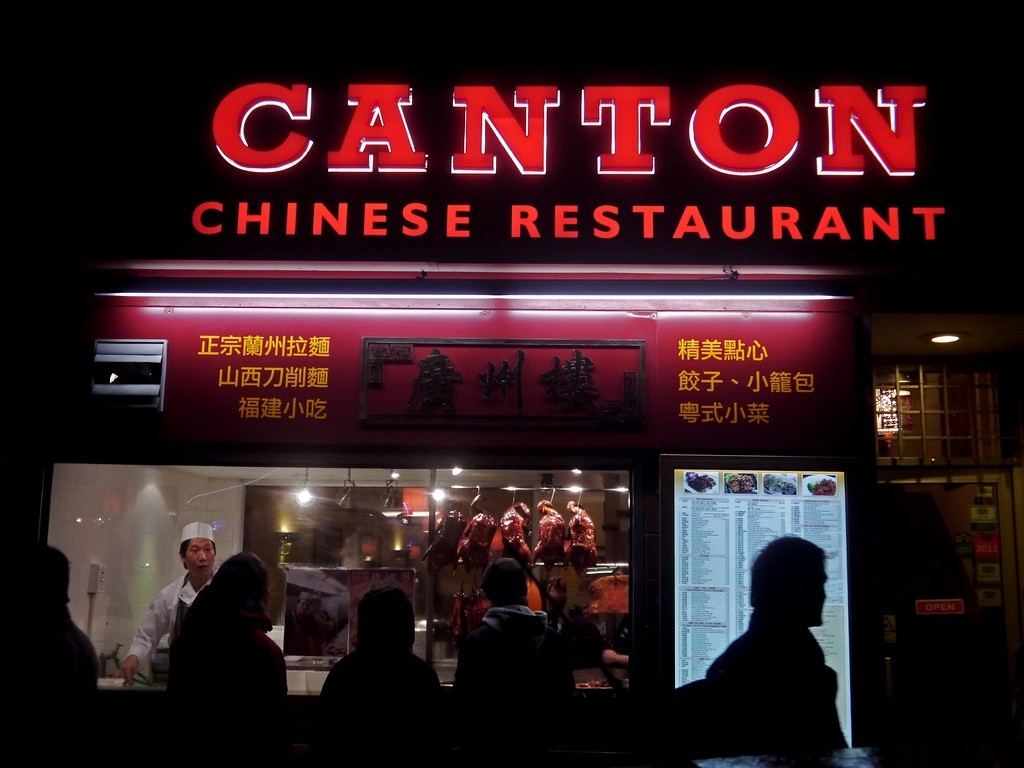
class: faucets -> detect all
[106,643,124,663]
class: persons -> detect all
[440,558,598,749]
[284,591,334,656]
[167,561,301,717]
[10,548,96,693]
[116,533,219,683]
[631,537,846,768]
[309,590,444,742]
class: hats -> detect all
[179,522,214,546]
[482,558,525,604]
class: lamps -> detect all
[338,469,357,511]
[380,469,403,508]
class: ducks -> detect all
[423,494,597,575]
